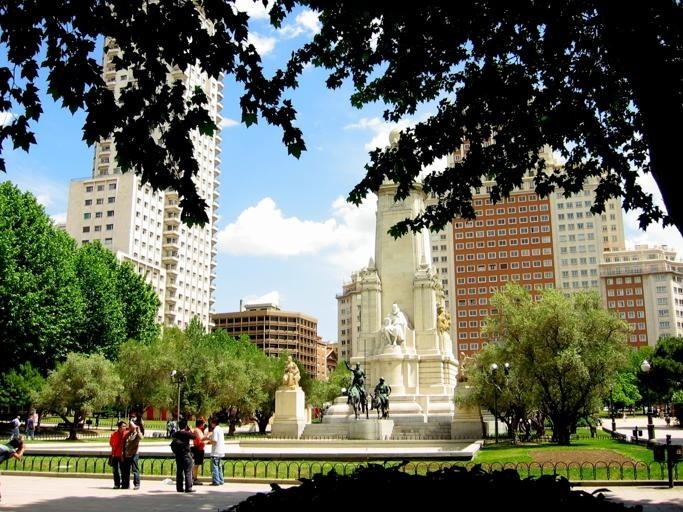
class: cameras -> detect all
[203,424,207,429]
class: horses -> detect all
[349,383,369,420]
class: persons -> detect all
[109,412,145,490]
[525,420,532,442]
[384,303,407,346]
[165,414,225,493]
[370,377,391,409]
[344,360,367,405]
[588,415,603,438]
[0,407,39,464]
[283,355,300,387]
[653,405,672,429]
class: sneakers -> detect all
[178,479,223,492]
[113,485,140,490]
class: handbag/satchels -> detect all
[191,447,198,453]
[171,438,185,455]
[9,422,15,429]
[108,455,113,466]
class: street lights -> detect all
[641,360,657,442]
[170,369,187,432]
[609,384,616,431]
[490,362,510,444]
